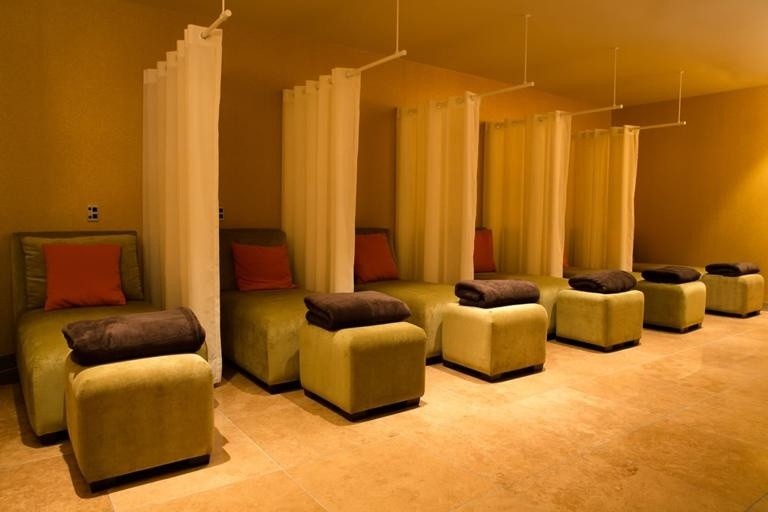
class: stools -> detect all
[554,290,646,353]
[297,321,423,420]
[440,302,549,383]
[62,354,213,491]
[639,281,707,333]
[704,274,765,318]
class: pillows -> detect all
[476,227,496,273]
[231,242,298,292]
[43,244,125,313]
[21,235,144,310]
[356,235,401,282]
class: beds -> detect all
[2,231,163,445]
[216,227,319,393]
[355,227,461,365]
[476,230,570,343]
[634,262,705,281]
[563,266,642,294]
